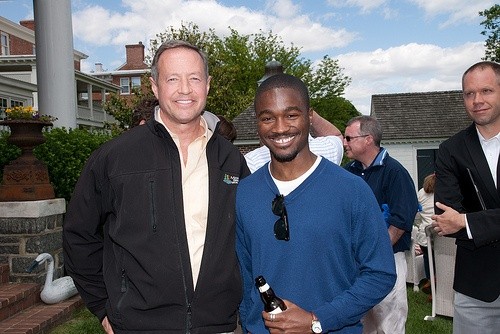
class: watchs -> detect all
[311,312,323,334]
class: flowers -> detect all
[5,105,59,123]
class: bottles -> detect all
[255,276,287,321]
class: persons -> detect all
[63,40,251,334]
[133,114,436,334]
[431,62,500,334]
[235,74,397,334]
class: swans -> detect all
[28,252,78,305]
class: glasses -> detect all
[344,135,370,142]
[271,194,290,241]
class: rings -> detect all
[270,313,276,322]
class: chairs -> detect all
[423,225,457,322]
[405,227,426,292]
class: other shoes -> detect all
[422,285,431,293]
